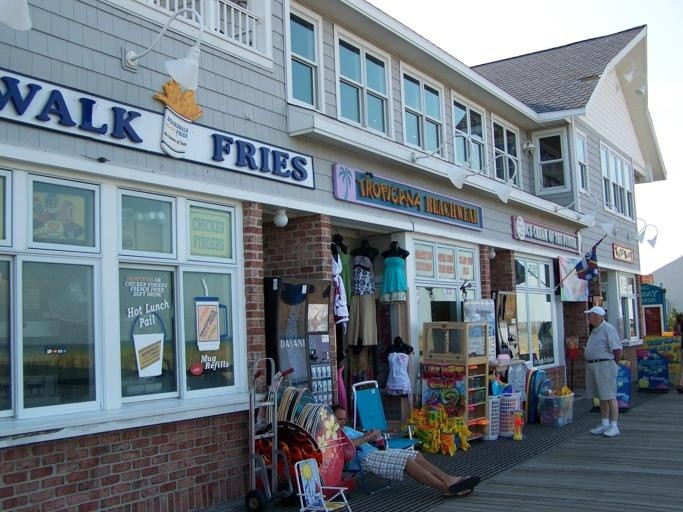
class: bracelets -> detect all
[367,436,370,441]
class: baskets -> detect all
[483,392,519,441]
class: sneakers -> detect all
[591,426,620,437]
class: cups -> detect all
[320,351,329,360]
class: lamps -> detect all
[466,153,518,203]
[121,6,204,90]
[627,224,657,247]
[597,202,617,236]
[487,247,495,259]
[616,72,649,97]
[272,206,288,227]
[607,59,635,83]
[553,191,599,228]
[410,133,474,190]
[617,218,645,243]
[460,278,471,300]
[522,140,536,153]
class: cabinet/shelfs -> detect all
[422,322,488,444]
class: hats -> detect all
[584,306,605,317]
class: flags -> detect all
[576,240,604,283]
[515,259,528,284]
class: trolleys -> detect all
[246,357,297,510]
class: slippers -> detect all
[443,476,479,498]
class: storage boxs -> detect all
[539,390,575,430]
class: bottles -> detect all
[373,432,386,451]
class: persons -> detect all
[380,240,409,302]
[332,404,480,498]
[338,353,347,408]
[331,243,349,323]
[348,239,379,345]
[584,306,622,437]
[346,339,373,395]
[386,336,414,396]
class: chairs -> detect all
[342,447,392,495]
[289,458,353,512]
[352,377,418,451]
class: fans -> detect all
[47,278,91,324]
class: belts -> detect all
[588,359,608,363]
